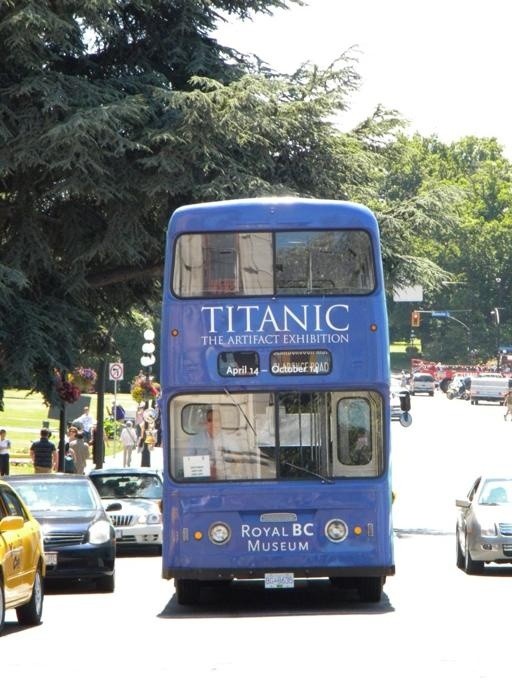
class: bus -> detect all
[157,191,419,606]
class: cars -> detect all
[388,376,407,418]
[402,355,511,409]
[85,465,162,556]
[452,471,511,575]
[0,480,49,631]
[1,473,122,592]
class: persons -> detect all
[0,430,11,477]
[504,391,512,422]
[30,401,162,474]
[186,408,242,468]
[400,370,408,390]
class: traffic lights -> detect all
[410,312,420,327]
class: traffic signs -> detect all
[433,310,449,317]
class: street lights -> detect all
[139,323,156,465]
[409,328,416,362]
